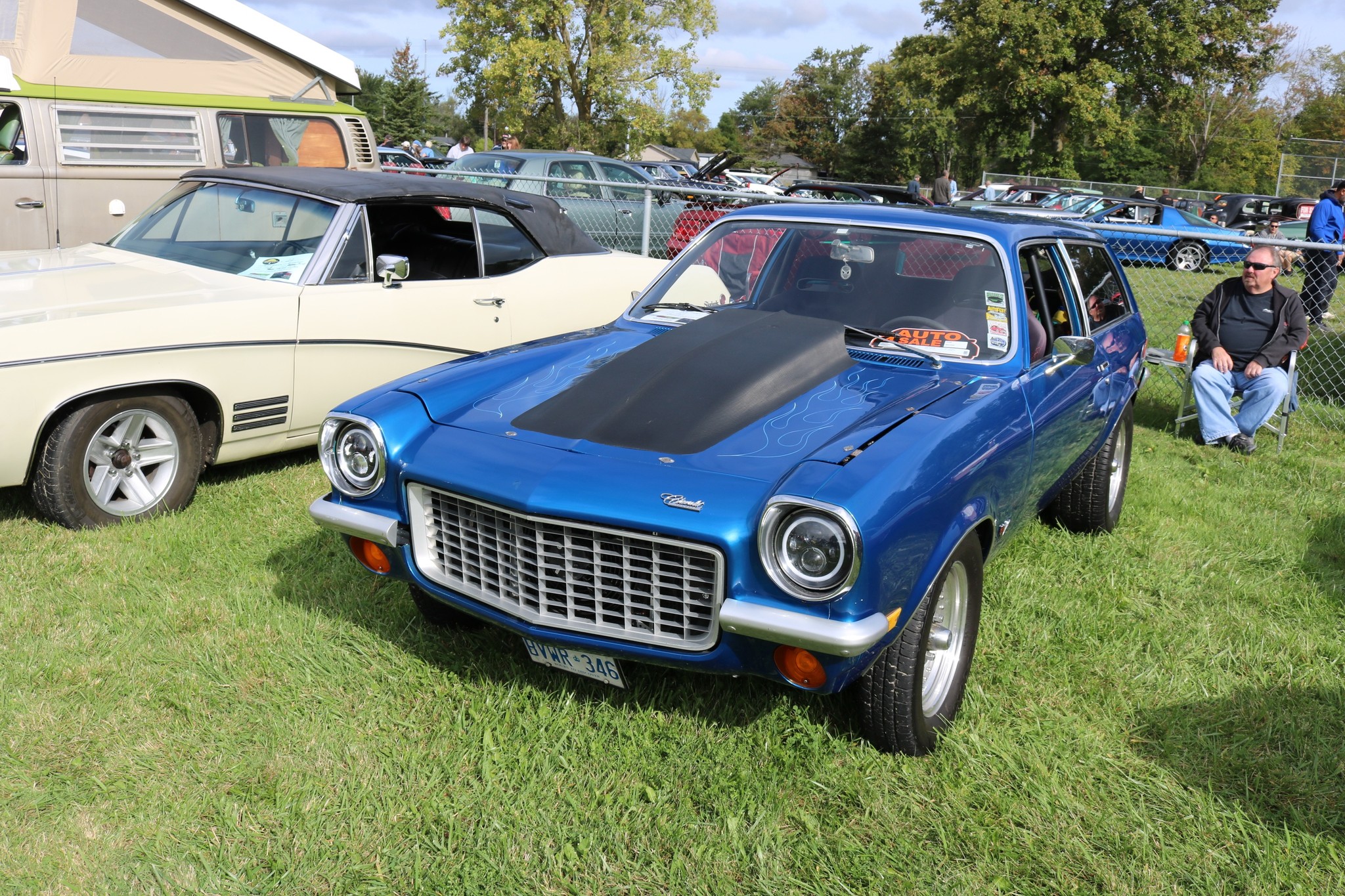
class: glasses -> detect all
[1270,225,1278,228]
[1209,217,1217,220]
[1243,261,1276,270]
[501,139,506,142]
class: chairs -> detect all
[1173,339,1297,454]
[547,166,567,195]
[0,105,22,165]
[562,169,591,197]
[920,265,1047,364]
[758,255,877,327]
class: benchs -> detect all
[391,224,527,278]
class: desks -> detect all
[1145,348,1189,389]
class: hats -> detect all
[914,174,922,178]
[1134,186,1143,191]
[413,140,422,147]
[499,133,511,140]
[384,135,392,140]
[401,141,410,147]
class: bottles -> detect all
[1172,320,1190,363]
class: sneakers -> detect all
[1321,312,1335,319]
[1308,321,1334,332]
[1194,431,1257,457]
[1305,315,1311,321]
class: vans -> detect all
[0,53,382,252]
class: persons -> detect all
[1081,296,1142,419]
[907,170,995,207]
[1128,180,1345,332]
[1192,245,1307,454]
[378,133,518,181]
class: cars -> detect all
[0,137,731,534]
[307,203,1151,756]
[377,146,1344,276]
